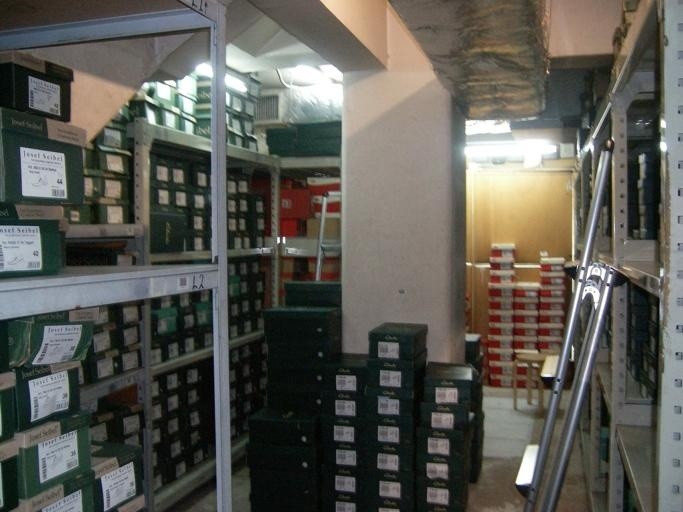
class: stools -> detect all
[513,352,547,416]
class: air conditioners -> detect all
[254,90,286,126]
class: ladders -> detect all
[315,192,342,282]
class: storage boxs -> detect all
[195,73,258,152]
[150,144,266,253]
[142,71,198,135]
[278,175,341,240]
[487,243,566,391]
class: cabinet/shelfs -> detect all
[592,93,615,505]
[613,0,683,512]
[128,119,279,511]
[0,0,225,512]
[271,154,341,259]
[71,109,143,509]
